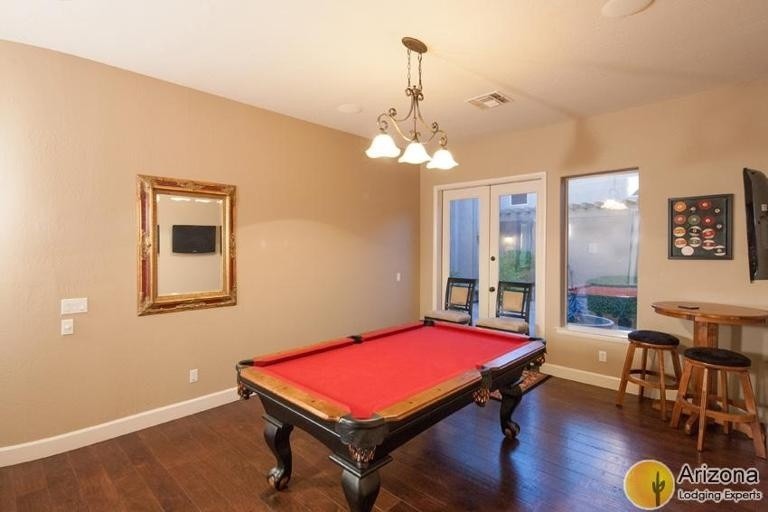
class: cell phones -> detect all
[679,305,699,309]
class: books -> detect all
[678,304,699,309]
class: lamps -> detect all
[601,177,629,211]
[364,37,460,172]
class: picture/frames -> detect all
[667,194,732,260]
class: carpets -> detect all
[487,369,551,403]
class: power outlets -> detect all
[190,369,198,384]
[598,350,607,363]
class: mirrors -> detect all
[136,173,239,318]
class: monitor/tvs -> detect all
[172,225,216,253]
[743,168,768,282]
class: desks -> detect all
[650,301,768,428]
[233,319,548,512]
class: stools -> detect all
[669,346,766,459]
[616,330,681,421]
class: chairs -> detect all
[475,279,535,336]
[422,276,475,327]
